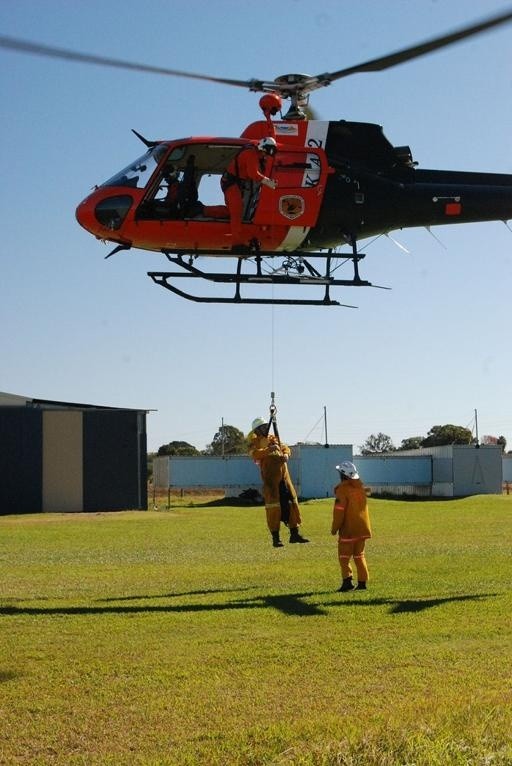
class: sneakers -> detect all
[231,244,253,251]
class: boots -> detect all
[272,531,284,547]
[289,527,309,543]
[337,576,354,592]
[355,581,366,591]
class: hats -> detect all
[336,461,360,480]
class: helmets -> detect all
[257,137,276,156]
[252,418,268,432]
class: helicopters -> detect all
[0,6,512,309]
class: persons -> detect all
[247,416,309,547]
[220,137,278,225]
[330,459,372,592]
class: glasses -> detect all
[263,144,276,153]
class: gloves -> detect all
[261,176,278,190]
